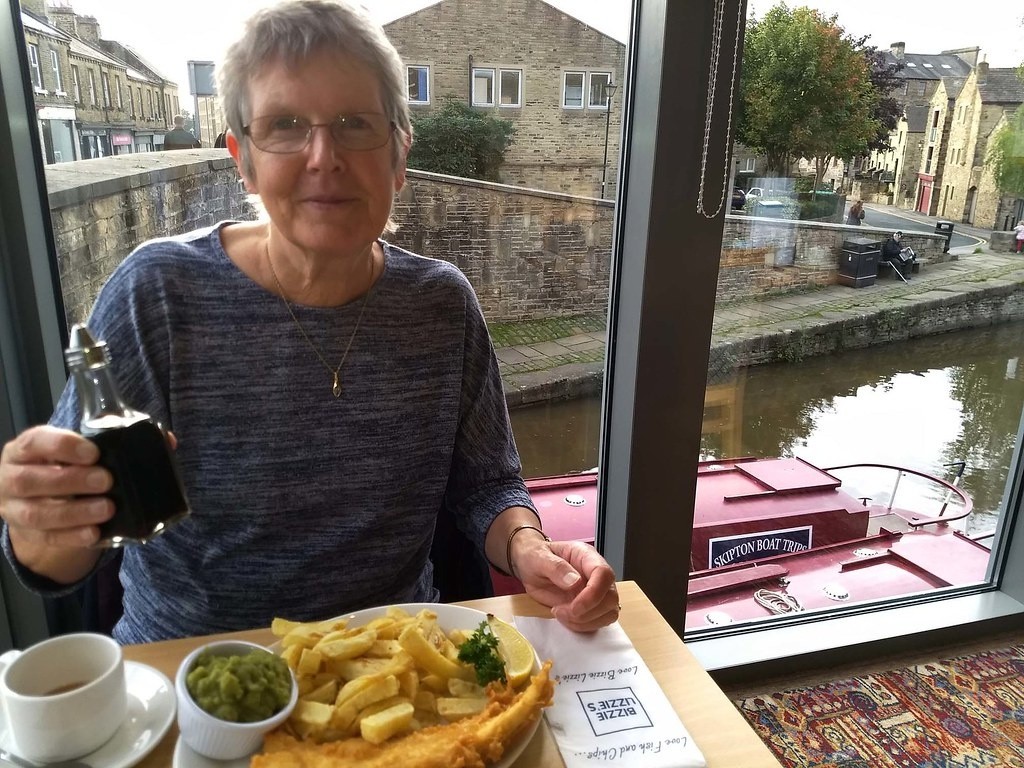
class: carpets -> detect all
[734,643,1024,767]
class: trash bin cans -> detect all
[935,221,954,254]
[838,237,880,288]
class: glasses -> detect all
[242,111,398,154]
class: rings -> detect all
[618,604,622,610]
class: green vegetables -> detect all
[457,622,508,687]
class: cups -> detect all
[0,632,129,764]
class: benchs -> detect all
[878,257,928,279]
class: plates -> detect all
[170,602,545,767]
[0,658,176,768]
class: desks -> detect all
[121,579,784,768]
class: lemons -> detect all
[486,613,534,687]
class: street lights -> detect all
[601,78,618,199]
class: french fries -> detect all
[270,607,496,745]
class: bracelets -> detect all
[507,525,552,587]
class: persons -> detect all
[846,201,865,226]
[214,125,229,148]
[884,231,913,281]
[1014,220,1024,253]
[164,115,202,151]
[1,0,619,649]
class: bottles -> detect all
[65,323,193,549]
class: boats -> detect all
[482,451,993,633]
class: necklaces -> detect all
[266,239,375,398]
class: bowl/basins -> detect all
[173,639,299,761]
